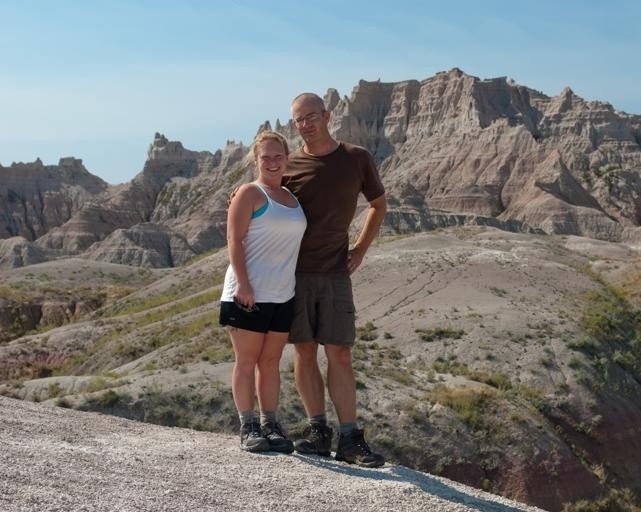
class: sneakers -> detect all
[239,420,268,450]
[336,429,384,468]
[295,424,333,456]
[260,421,295,453]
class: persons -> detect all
[218,130,310,454]
[226,92,389,469]
